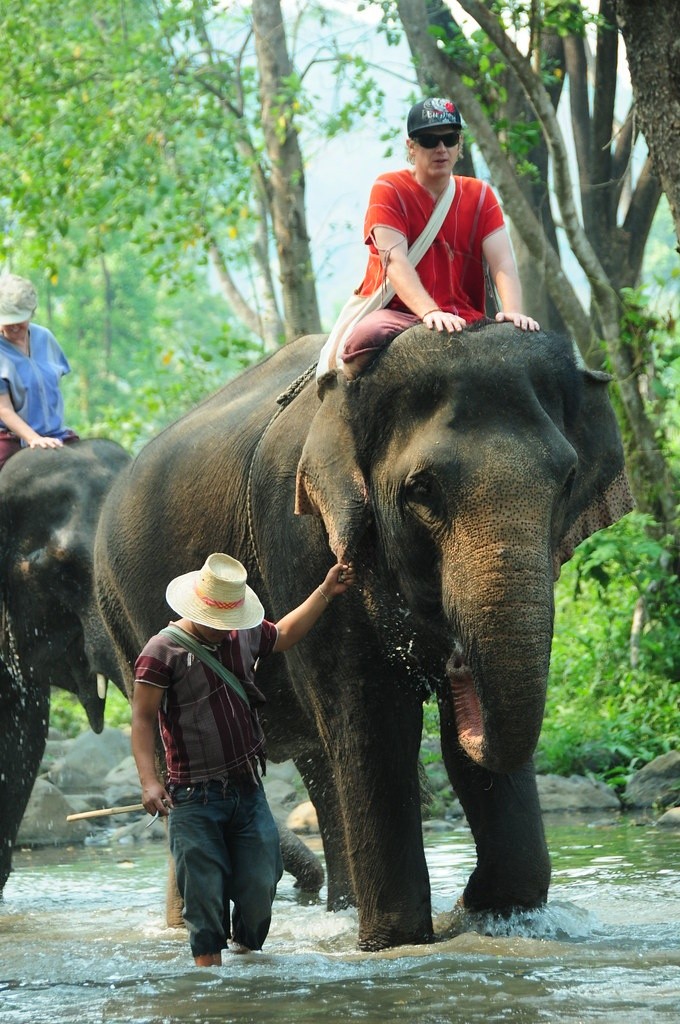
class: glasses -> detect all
[411,131,462,148]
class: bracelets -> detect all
[421,308,443,323]
[318,585,334,603]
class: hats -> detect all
[407,98,463,134]
[0,272,37,325]
[165,552,265,630]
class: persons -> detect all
[0,272,80,473]
[131,552,355,967]
[341,97,541,385]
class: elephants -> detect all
[0,320,636,950]
